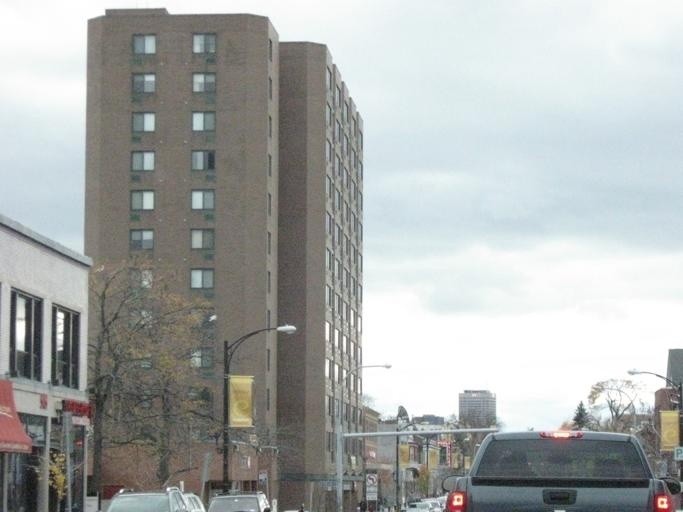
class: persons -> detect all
[355,495,388,512]
[298,503,305,512]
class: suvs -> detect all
[109,487,269,511]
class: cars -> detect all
[445,429,682,511]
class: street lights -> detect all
[340,364,391,449]
[395,421,481,511]
[224,325,296,492]
[628,370,682,442]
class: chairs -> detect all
[591,458,627,479]
[494,450,533,476]
